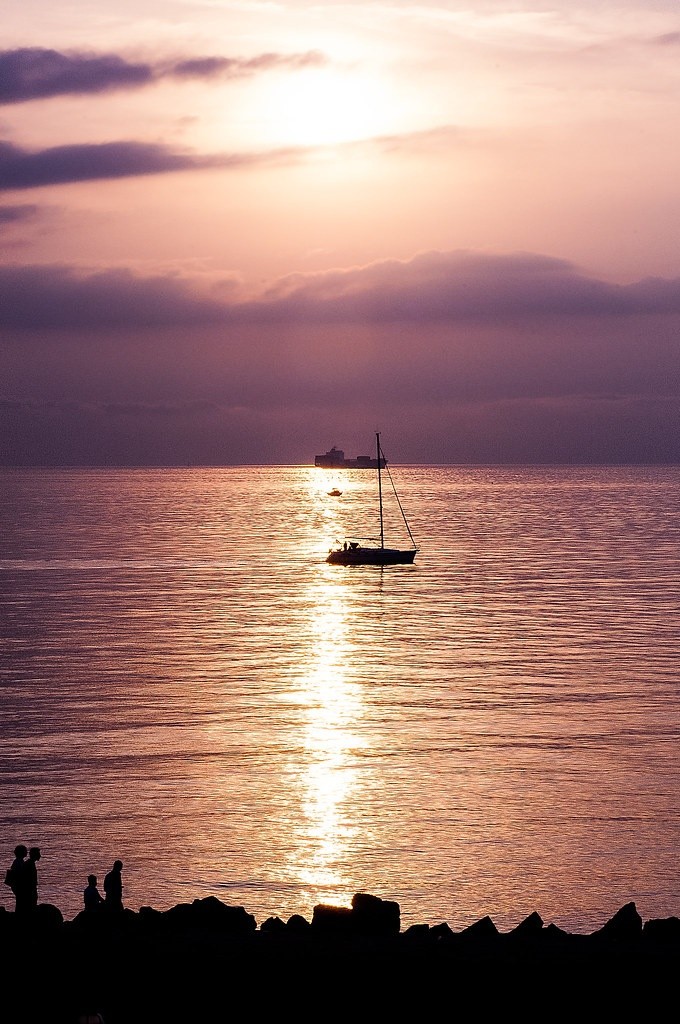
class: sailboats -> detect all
[326,433,420,565]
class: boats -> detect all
[315,447,388,470]
[327,491,342,496]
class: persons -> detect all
[5,844,42,912]
[103,860,124,912]
[83,875,105,910]
